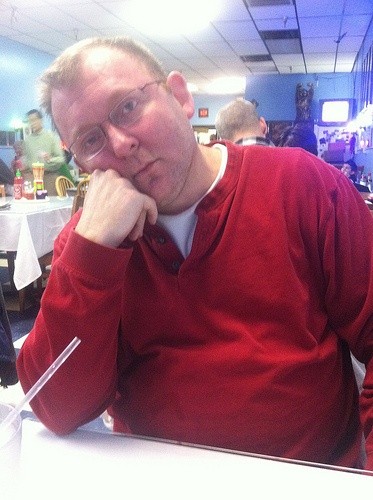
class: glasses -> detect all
[69,80,166,163]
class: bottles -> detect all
[14,169,23,199]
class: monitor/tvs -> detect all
[318,98,357,127]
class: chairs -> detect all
[77,180,95,195]
[18,194,84,312]
[55,176,75,197]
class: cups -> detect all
[0,403,22,457]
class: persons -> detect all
[270,123,318,160]
[12,36,372,474]
[214,96,273,150]
[0,159,15,197]
[18,109,66,197]
[11,140,24,175]
[340,160,358,187]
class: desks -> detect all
[67,186,87,197]
[0,197,77,311]
[0,419,373,500]
